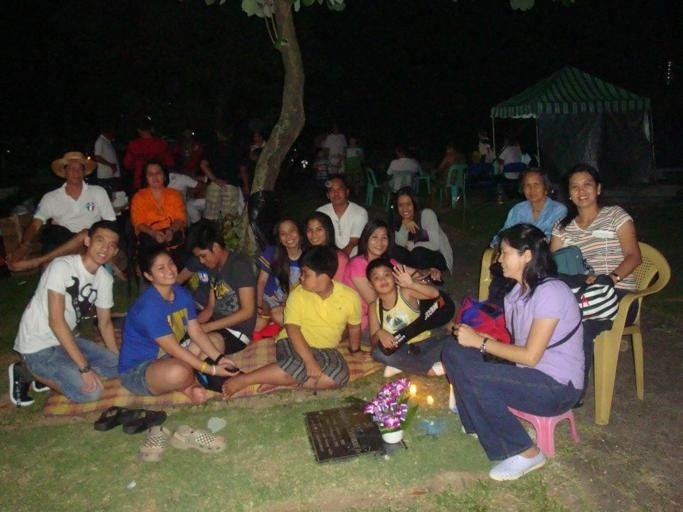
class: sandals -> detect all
[170,425,227,453]
[140,426,172,463]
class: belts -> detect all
[224,325,252,346]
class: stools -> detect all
[504,406,582,460]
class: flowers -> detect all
[346,375,417,435]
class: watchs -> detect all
[78,364,92,374]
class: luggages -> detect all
[454,295,513,346]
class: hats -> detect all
[50,151,98,179]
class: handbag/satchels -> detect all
[137,216,184,248]
[551,245,595,278]
[570,282,621,322]
[483,349,516,368]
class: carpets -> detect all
[40,328,385,417]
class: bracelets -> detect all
[608,271,622,283]
[214,353,224,364]
[200,361,207,372]
[480,337,488,354]
[347,347,361,353]
[211,363,215,377]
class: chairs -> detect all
[591,239,670,426]
[119,206,183,293]
[341,153,525,217]
[477,247,496,301]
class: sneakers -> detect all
[384,364,403,377]
[430,360,447,376]
[488,448,547,482]
[32,378,51,393]
[8,361,35,406]
[460,423,478,440]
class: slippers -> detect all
[93,406,130,431]
[122,408,167,434]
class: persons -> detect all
[222,246,362,400]
[175,254,212,307]
[253,218,306,333]
[6,221,120,408]
[366,258,458,377]
[306,210,350,342]
[190,225,255,357]
[439,223,585,483]
[488,169,568,301]
[342,220,442,349]
[548,164,642,407]
[115,249,240,405]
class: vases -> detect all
[381,430,404,443]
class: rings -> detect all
[390,340,394,343]
